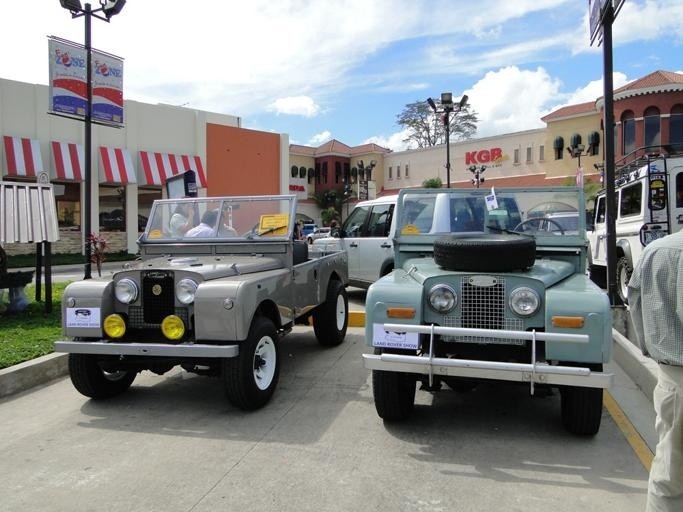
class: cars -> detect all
[537,211,579,237]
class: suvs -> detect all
[306,192,525,290]
[584,155,683,306]
[361,187,613,436]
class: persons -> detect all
[208,207,238,238]
[168,195,195,238]
[625,225,683,511]
[181,209,216,238]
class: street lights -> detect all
[553,131,599,184]
[426,92,468,186]
[341,160,376,200]
[468,163,486,188]
[58,0,125,279]
